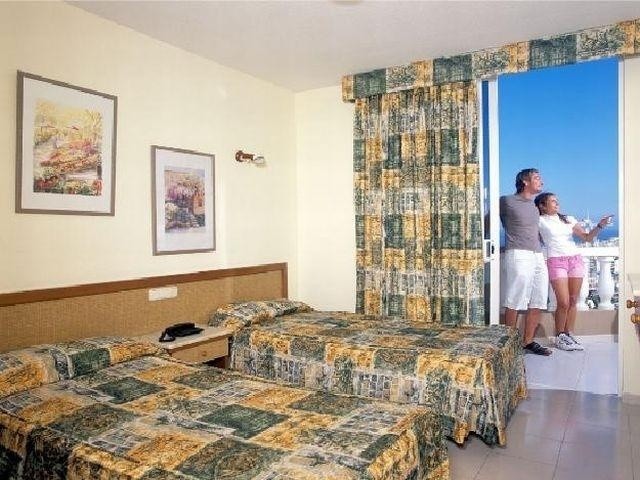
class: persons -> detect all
[535,193,616,351]
[484,168,553,356]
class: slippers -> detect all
[522,342,552,356]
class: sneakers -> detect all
[568,332,584,350]
[555,333,575,351]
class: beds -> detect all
[211,300,528,448]
[0,335,451,480]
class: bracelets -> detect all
[597,224,603,230]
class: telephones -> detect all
[158,323,204,342]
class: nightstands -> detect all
[133,323,233,364]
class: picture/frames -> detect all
[151,146,215,255]
[14,70,115,217]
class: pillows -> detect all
[211,301,313,329]
[3,337,165,396]
